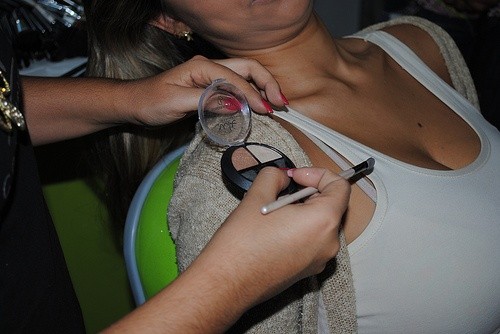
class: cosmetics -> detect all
[198,79,298,201]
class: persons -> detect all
[84,0,500,334]
[0,47,352,334]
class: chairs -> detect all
[123,143,190,308]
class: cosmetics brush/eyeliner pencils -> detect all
[260,158,375,214]
